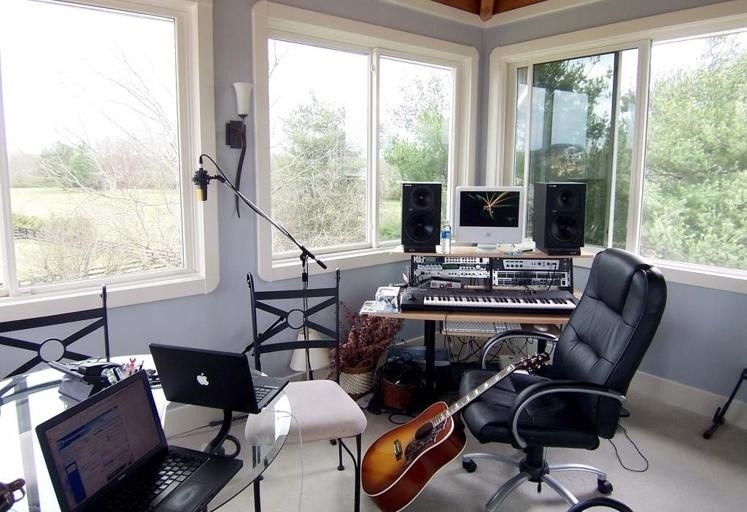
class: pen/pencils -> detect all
[113,358,144,381]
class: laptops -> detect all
[148,342,291,415]
[34,368,244,512]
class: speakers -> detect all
[401,181,443,253]
[533,181,587,256]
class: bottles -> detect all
[440,222,451,254]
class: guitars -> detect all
[362,350,549,512]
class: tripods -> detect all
[209,174,337,446]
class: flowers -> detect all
[326,298,407,383]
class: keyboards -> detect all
[442,321,523,334]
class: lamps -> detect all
[289,328,330,380]
[224,82,253,220]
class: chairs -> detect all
[245,268,368,512]
[0,284,110,383]
[458,246,667,512]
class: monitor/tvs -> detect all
[455,185,527,250]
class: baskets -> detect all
[340,358,375,396]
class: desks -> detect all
[0,352,294,512]
[359,246,570,402]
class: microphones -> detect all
[194,156,208,202]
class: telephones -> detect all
[47,357,121,382]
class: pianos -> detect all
[400,286,578,315]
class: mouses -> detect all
[534,324,550,333]
